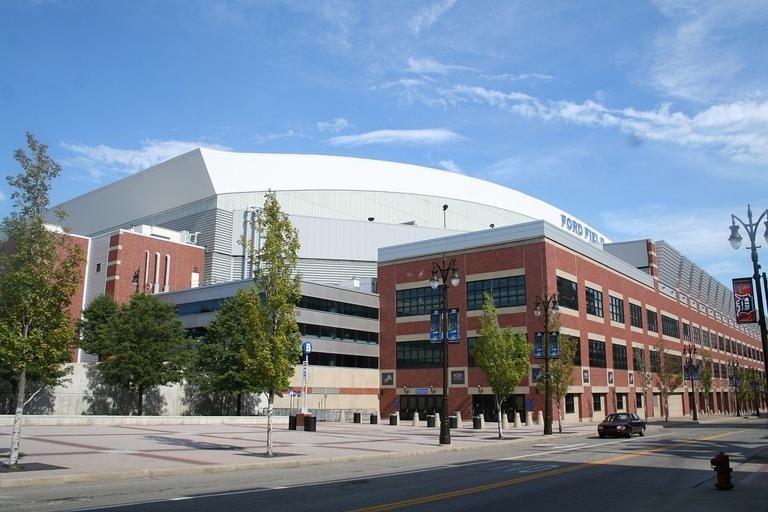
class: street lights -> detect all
[726,201,768,399]
[429,250,462,445]
[131,266,143,296]
[726,357,742,416]
[746,366,761,418]
[681,342,701,420]
[533,292,562,435]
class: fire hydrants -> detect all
[709,450,736,491]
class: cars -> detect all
[595,410,649,440]
